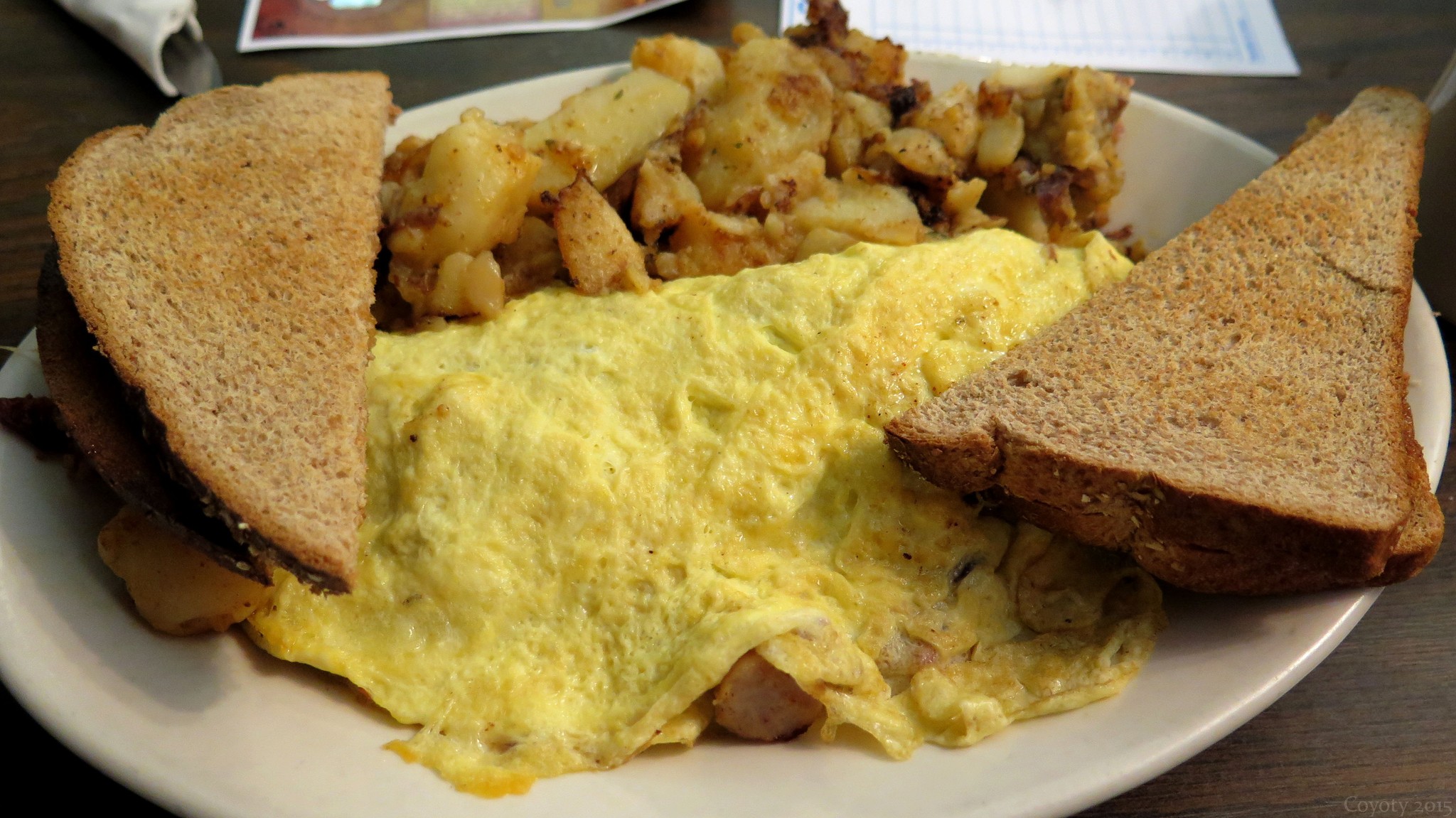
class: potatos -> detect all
[379,0,1137,323]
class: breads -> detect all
[881,85,1428,578]
[46,69,395,594]
[954,108,1442,594]
[37,242,277,584]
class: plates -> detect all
[0,58,1452,817]
